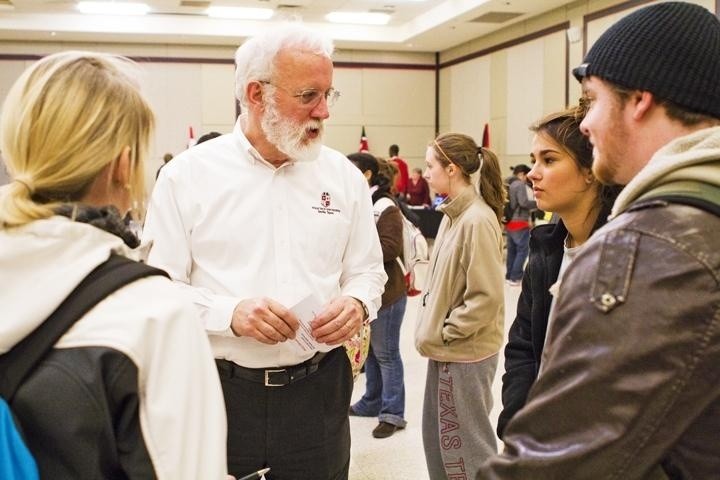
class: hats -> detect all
[570,1,720,120]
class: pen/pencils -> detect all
[239,466,273,480]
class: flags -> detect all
[359,126,368,152]
[483,124,489,147]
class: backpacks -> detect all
[372,196,431,298]
[0,251,174,480]
[501,176,521,226]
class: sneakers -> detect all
[347,405,360,417]
[371,420,406,438]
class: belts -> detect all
[213,350,330,389]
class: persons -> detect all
[476,2,720,480]
[498,99,622,440]
[0,50,228,480]
[141,23,389,480]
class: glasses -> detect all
[258,79,342,108]
